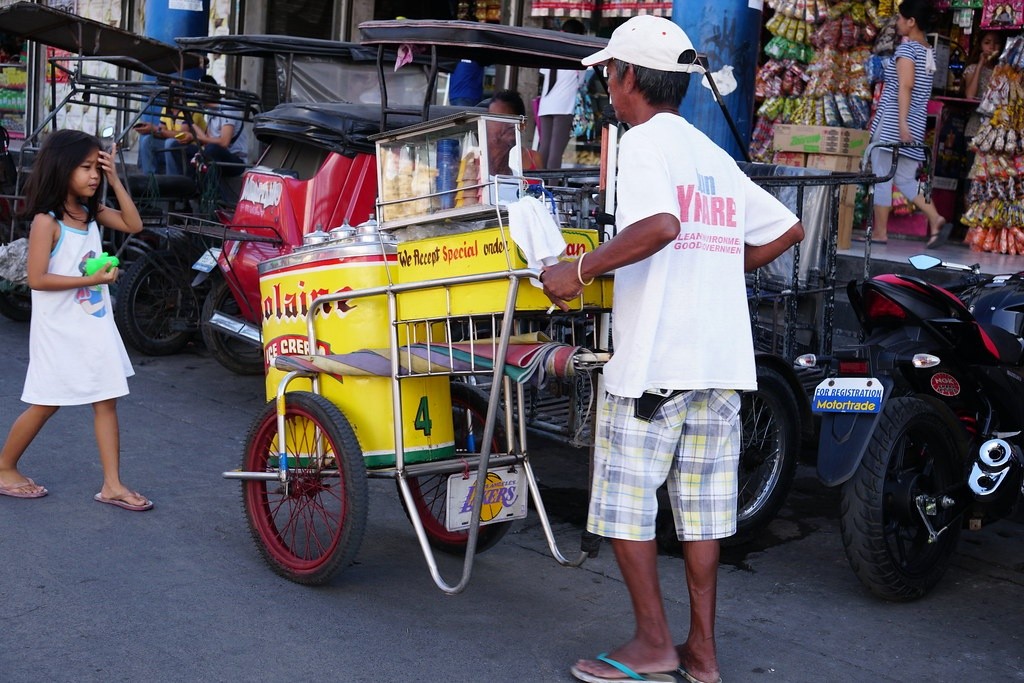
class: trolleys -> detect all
[223,108,615,596]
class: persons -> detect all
[0,128,154,512]
[490,89,542,183]
[961,30,1010,244]
[541,14,804,683]
[539,19,586,186]
[862,0,953,249]
[135,75,248,174]
[486,120,519,204]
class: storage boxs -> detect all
[933,177,959,223]
[396,224,598,320]
[772,124,864,180]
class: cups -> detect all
[435,139,459,210]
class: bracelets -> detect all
[578,252,595,285]
[157,127,162,135]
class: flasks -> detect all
[305,216,384,251]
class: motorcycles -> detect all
[810,255,1024,604]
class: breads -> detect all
[561,150,601,165]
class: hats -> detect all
[580,14,707,74]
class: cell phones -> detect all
[175,133,186,140]
[133,123,145,128]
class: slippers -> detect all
[851,236,888,245]
[926,222,952,249]
[676,662,722,683]
[94,491,153,510]
[570,651,676,683]
[0,477,48,497]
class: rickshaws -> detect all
[0,0,932,551]
